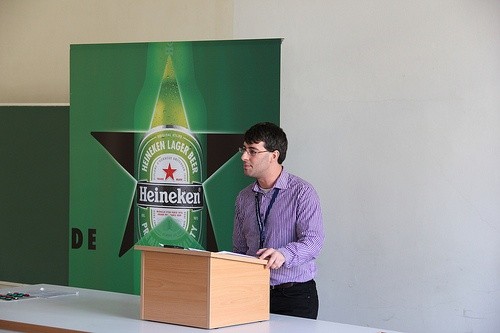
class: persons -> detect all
[233,122,324,320]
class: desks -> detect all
[0,281,406,333]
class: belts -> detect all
[270,282,293,290]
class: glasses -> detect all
[239,146,274,156]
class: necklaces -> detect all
[254,189,280,248]
